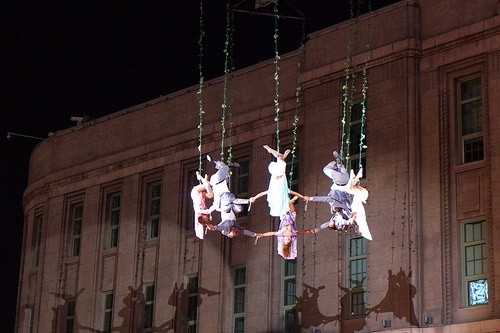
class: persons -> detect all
[203,154,253,214]
[192,171,214,240]
[346,166,373,240]
[251,144,308,217]
[207,208,258,239]
[260,195,316,260]
[305,150,355,219]
[315,205,353,235]
